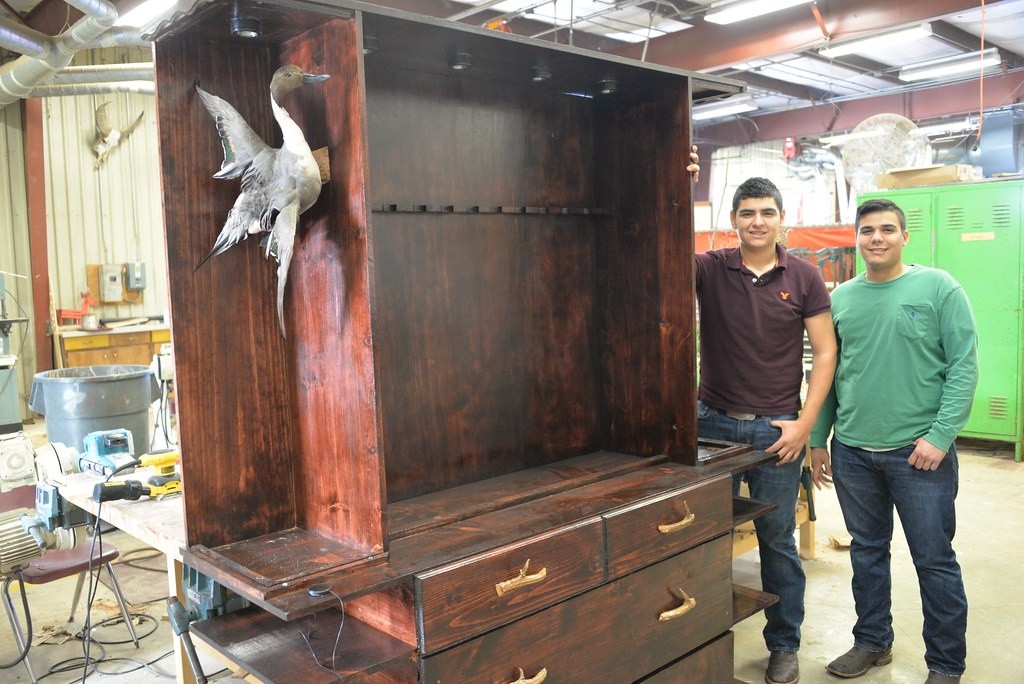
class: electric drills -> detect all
[92,447,184,503]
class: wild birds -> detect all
[192,64,331,341]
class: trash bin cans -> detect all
[28,365,162,462]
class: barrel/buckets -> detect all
[28,364,163,473]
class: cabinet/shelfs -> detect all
[154,0,781,684]
[856,178,1024,463]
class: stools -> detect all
[0,539,141,684]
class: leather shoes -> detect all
[765,649,800,684]
[924,669,961,684]
[826,642,892,678]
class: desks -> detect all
[54,447,197,684]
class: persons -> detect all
[810,199,980,684]
[686,141,838,684]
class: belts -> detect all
[703,402,762,421]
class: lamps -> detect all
[703,0,814,26]
[694,91,760,122]
[819,22,933,59]
[898,46,1003,81]
